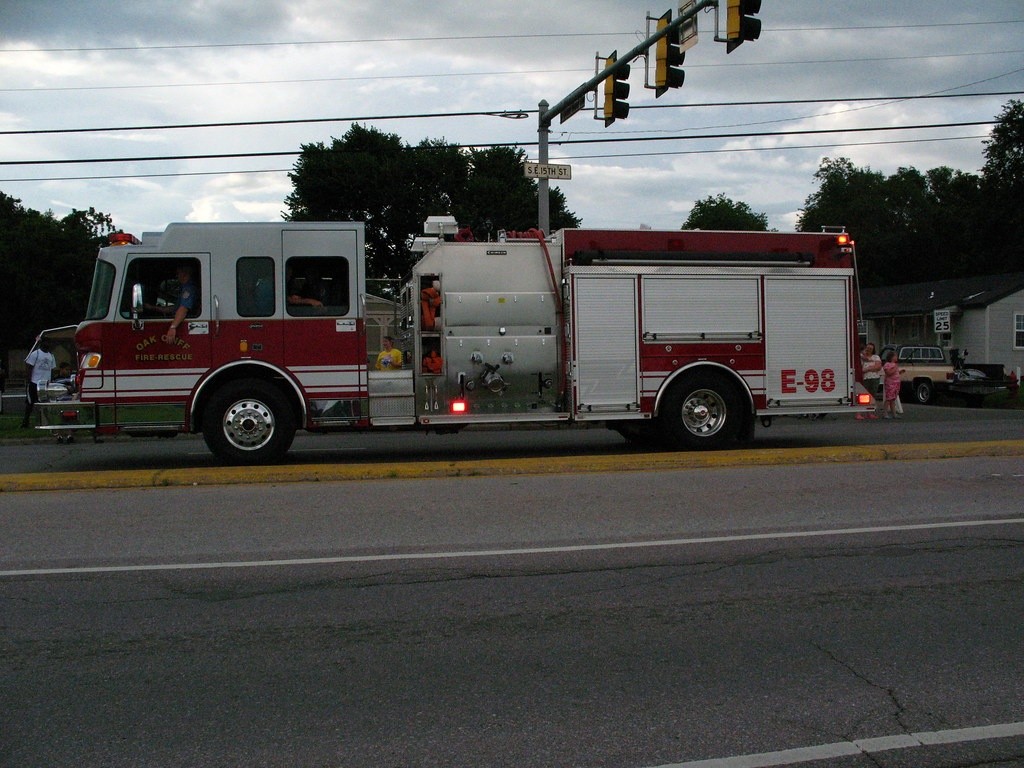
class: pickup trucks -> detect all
[879,343,1015,405]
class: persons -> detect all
[143,263,197,344]
[883,352,906,418]
[52,362,77,444]
[20,335,56,427]
[376,337,402,370]
[855,343,883,420]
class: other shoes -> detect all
[893,414,903,419]
[56,434,64,445]
[66,434,75,444]
[881,414,892,420]
[854,413,864,420]
[865,413,879,419]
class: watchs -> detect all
[170,325,176,329]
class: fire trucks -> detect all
[24,215,871,467]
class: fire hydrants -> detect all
[1005,370,1021,400]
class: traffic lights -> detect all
[727,0,762,54]
[604,50,630,128]
[656,8,686,98]
